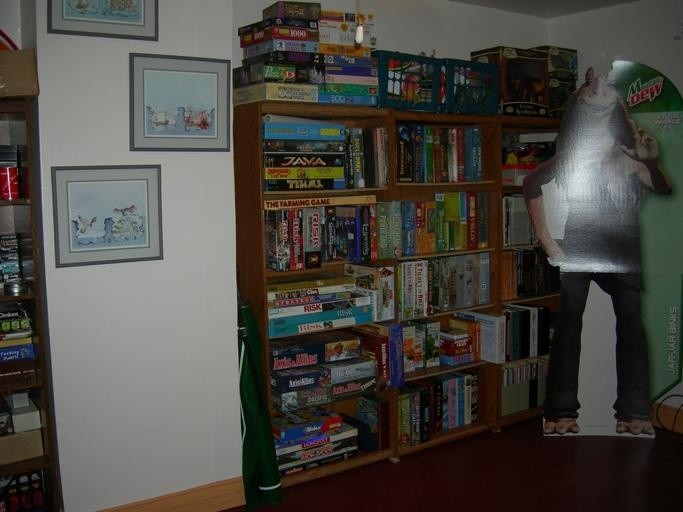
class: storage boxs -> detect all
[469,46,577,118]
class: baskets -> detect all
[443,58,501,115]
[372,50,444,113]
[0,467,57,512]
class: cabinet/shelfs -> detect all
[231,101,563,489]
[0,96,63,512]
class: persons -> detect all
[525,118,675,434]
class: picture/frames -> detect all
[44,0,231,269]
